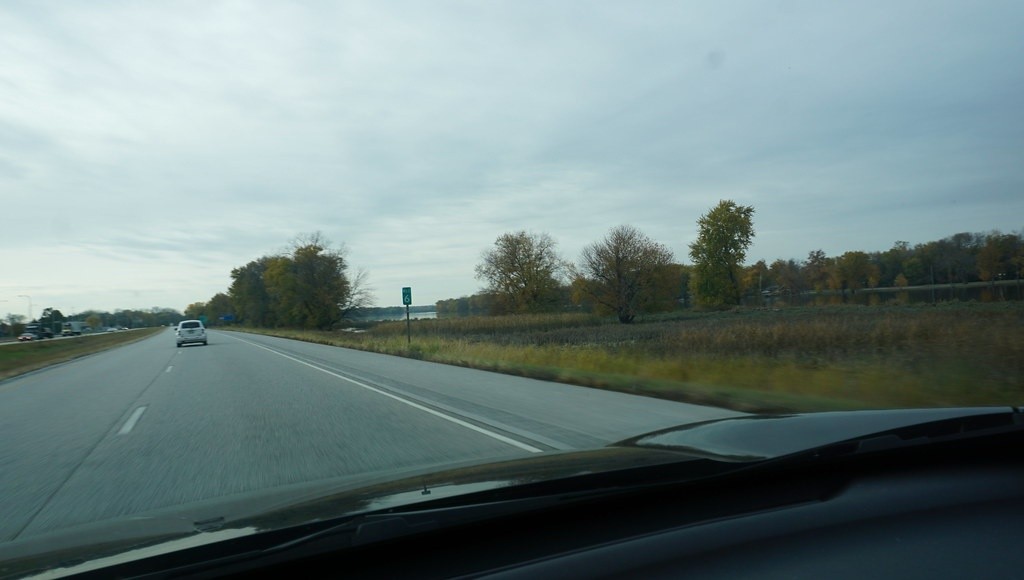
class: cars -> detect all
[26,321,85,339]
[108,327,129,332]
[18,332,39,341]
[174,320,208,347]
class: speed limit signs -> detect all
[402,287,412,305]
